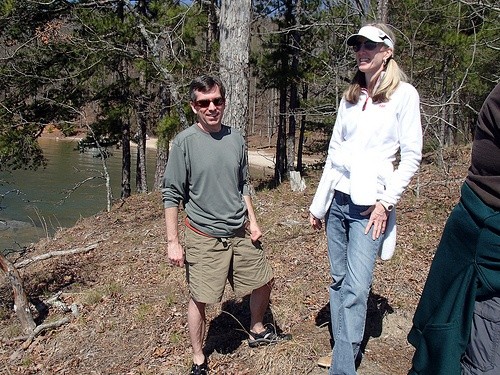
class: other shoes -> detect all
[318,351,333,367]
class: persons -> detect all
[305,22,424,375]
[404,79,500,375]
[161,74,293,375]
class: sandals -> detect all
[189,348,207,375]
[248,327,292,348]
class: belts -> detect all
[185,216,221,238]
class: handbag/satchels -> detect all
[378,204,396,261]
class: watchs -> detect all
[380,200,394,211]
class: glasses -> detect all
[196,98,224,108]
[352,40,379,53]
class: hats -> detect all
[346,25,394,53]
